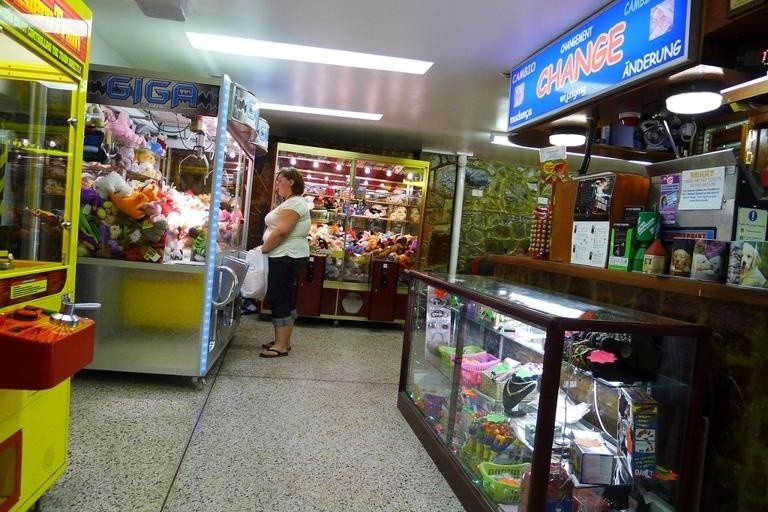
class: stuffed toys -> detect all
[42,98,247,268]
[278,151,420,284]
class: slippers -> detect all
[259,341,291,358]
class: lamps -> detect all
[548,125,587,152]
[661,85,723,117]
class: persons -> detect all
[257,165,311,359]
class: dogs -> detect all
[671,249,690,273]
[741,242,766,287]
[694,252,722,276]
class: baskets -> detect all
[479,462,532,505]
[439,345,501,386]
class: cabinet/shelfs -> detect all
[395,268,711,512]
[258,141,431,330]
